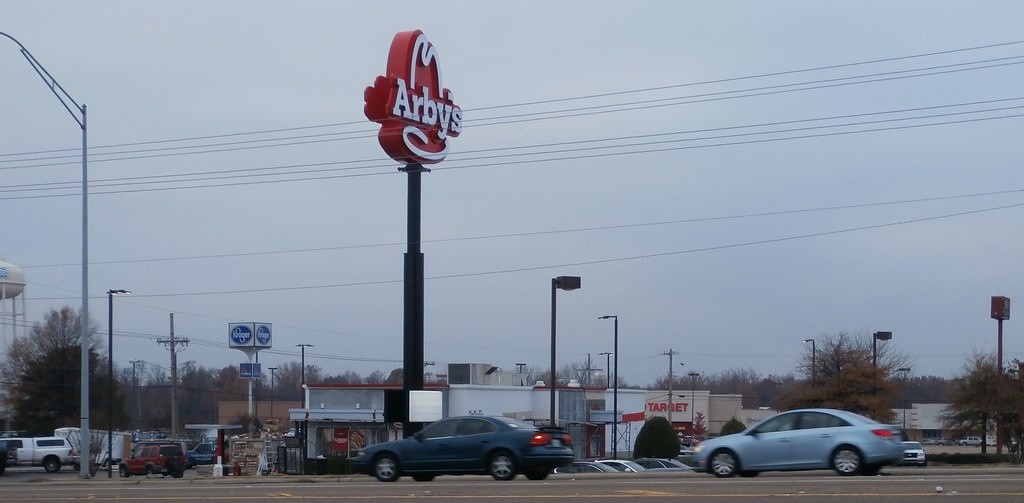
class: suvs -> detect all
[120,444,187,479]
[0,437,75,473]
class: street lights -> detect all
[899,367,911,430]
[598,315,617,458]
[802,339,815,384]
[268,367,278,419]
[295,344,315,409]
[550,276,583,425]
[872,332,891,394]
[107,290,131,477]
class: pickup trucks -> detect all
[0,446,18,467]
[187,442,229,469]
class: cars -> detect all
[679,435,699,456]
[936,437,957,446]
[693,408,905,477]
[920,439,936,446]
[352,415,576,482]
[552,457,693,472]
[958,437,982,446]
[986,435,997,445]
[901,441,927,468]
[132,431,165,442]
